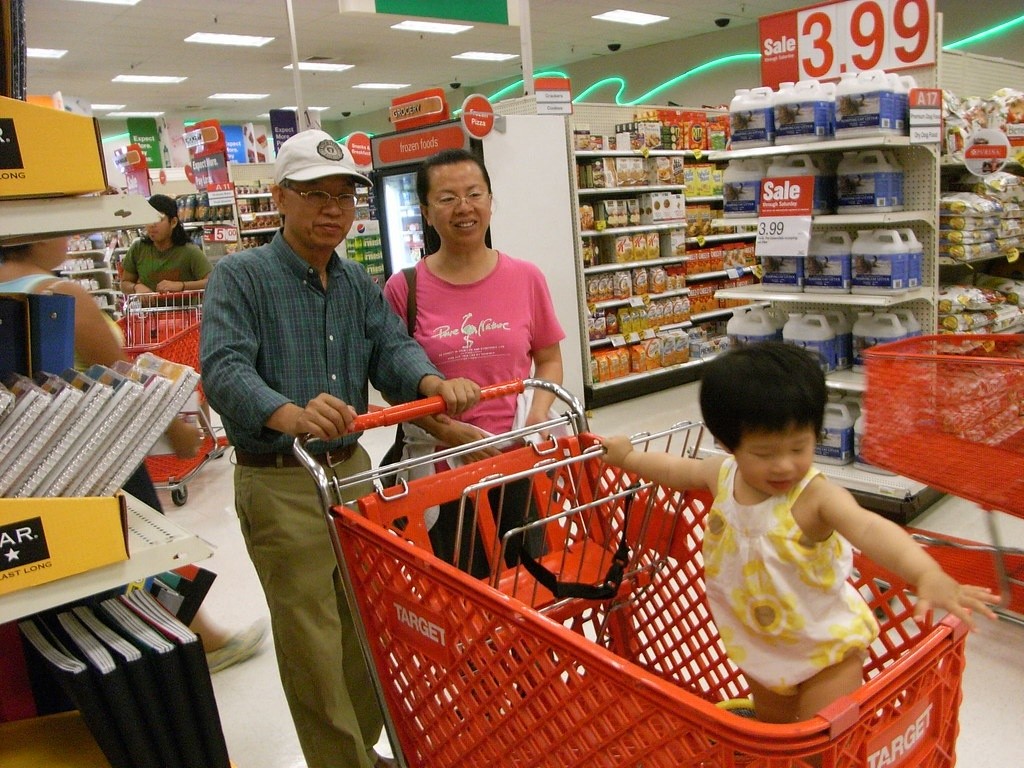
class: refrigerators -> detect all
[369,115,585,410]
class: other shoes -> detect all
[206,619,270,672]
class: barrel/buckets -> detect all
[813,403,854,465]
[782,313,835,374]
[852,312,907,373]
[806,310,851,370]
[724,70,923,295]
[828,393,862,419]
[727,308,776,343]
[854,408,898,477]
[873,309,921,337]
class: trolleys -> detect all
[110,289,237,507]
[854,329,1024,630]
[290,375,967,768]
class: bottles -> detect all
[746,306,784,341]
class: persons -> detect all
[596,340,1000,723]
[202,131,482,768]
[119,194,214,307]
[384,148,565,575]
[0,239,267,673]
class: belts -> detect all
[236,440,358,468]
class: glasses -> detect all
[281,185,359,209]
[425,191,491,208]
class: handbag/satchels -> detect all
[379,440,408,530]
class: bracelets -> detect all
[182,282,185,291]
[133,283,139,293]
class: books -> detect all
[0,352,228,768]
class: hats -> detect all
[274,129,373,187]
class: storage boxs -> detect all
[597,200,618,228]
[661,192,673,221]
[644,193,663,221]
[625,199,640,225]
[717,279,738,308]
[671,194,686,220]
[617,200,627,227]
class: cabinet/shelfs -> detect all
[0,0,1024,768]
